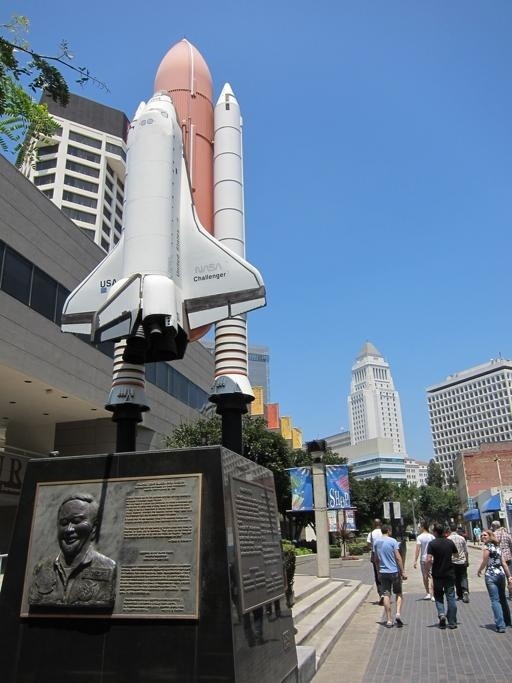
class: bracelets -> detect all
[506,573,511,576]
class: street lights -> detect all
[407,497,417,532]
[492,453,511,533]
[304,437,334,579]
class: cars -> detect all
[409,533,416,542]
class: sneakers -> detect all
[378,594,384,605]
[450,626,455,629]
[495,628,506,633]
[424,594,435,602]
[386,614,404,628]
[440,616,446,629]
[459,591,470,603]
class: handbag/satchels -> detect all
[371,551,374,562]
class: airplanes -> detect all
[54,84,274,371]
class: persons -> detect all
[28,491,117,613]
[413,521,436,601]
[392,521,511,579]
[366,517,394,605]
[264,597,281,621]
[372,523,408,627]
[477,527,511,633]
[491,519,511,601]
[228,548,266,647]
[445,521,471,603]
[424,522,460,629]
